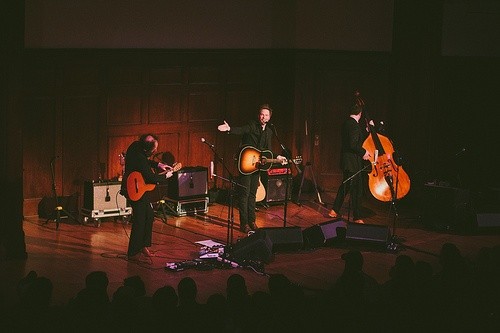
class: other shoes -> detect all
[250,223,258,230]
[354,220,365,224]
[144,246,156,257]
[328,209,345,218]
[128,255,150,263]
[239,224,252,233]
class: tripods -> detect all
[153,182,178,222]
[45,155,83,232]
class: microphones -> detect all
[105,188,110,201]
[456,148,466,156]
[189,175,193,188]
[265,121,274,126]
[210,161,214,180]
[201,138,215,148]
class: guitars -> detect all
[121,162,183,201]
[256,175,266,202]
[236,144,303,176]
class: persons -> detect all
[14,243,500,333]
[218,104,288,234]
[120,135,174,262]
[328,103,373,225]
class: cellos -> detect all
[354,89,411,215]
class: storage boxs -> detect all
[162,197,209,216]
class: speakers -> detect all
[303,218,347,248]
[256,226,303,251]
[167,166,208,200]
[233,230,272,265]
[266,177,292,203]
[84,181,126,210]
[344,221,390,252]
